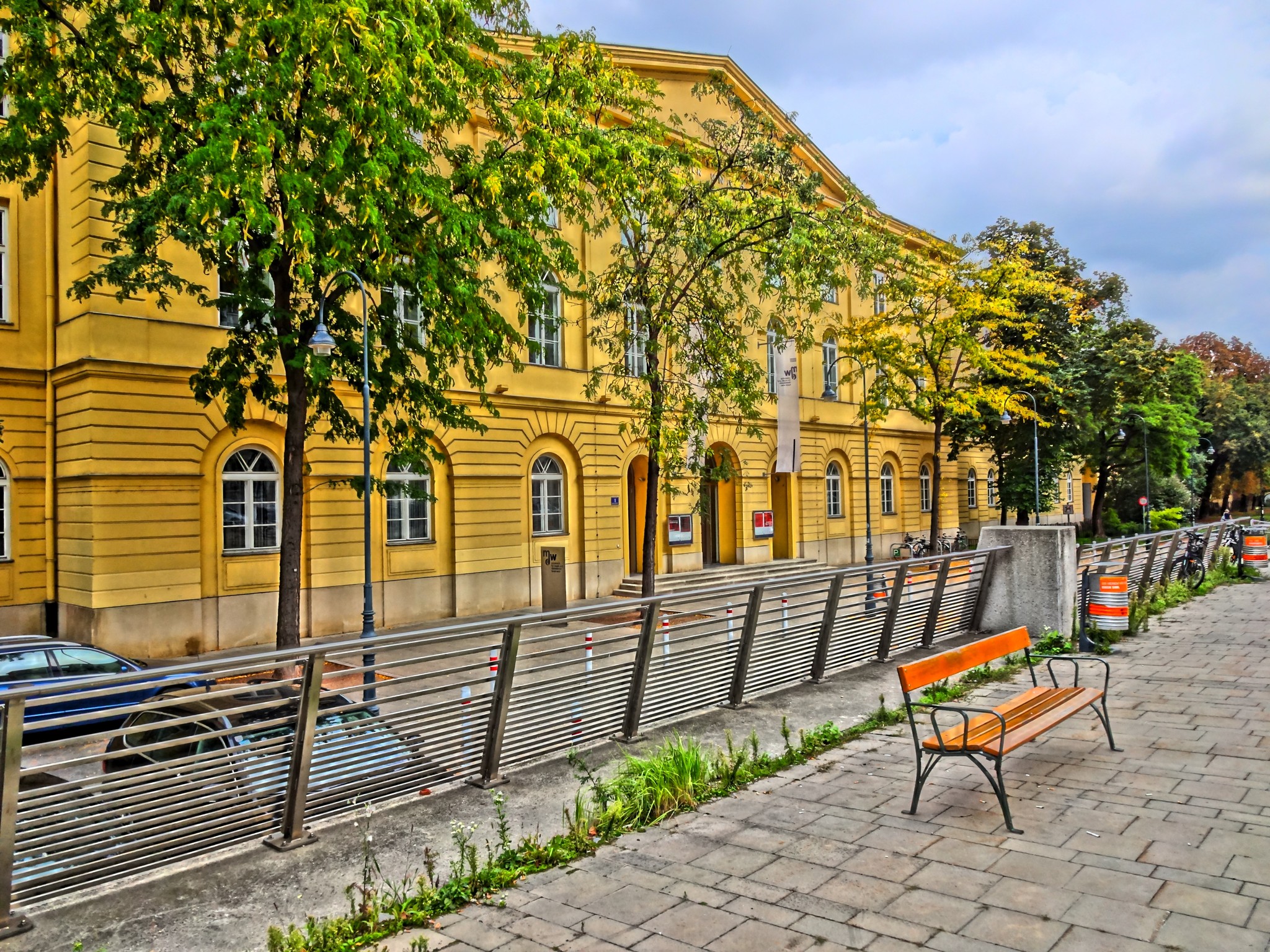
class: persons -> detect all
[1223,509,1235,532]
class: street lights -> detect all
[305,272,384,721]
[1190,438,1215,526]
[820,355,879,617]
[998,391,1042,527]
[1116,413,1153,554]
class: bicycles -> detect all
[1219,518,1251,569]
[894,528,968,562]
[1167,530,1208,591]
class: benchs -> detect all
[896,625,1124,834]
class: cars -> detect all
[101,679,454,860]
[0,635,220,746]
[0,766,198,905]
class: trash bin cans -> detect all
[1089,573,1130,630]
[872,573,887,602]
[1243,535,1268,567]
